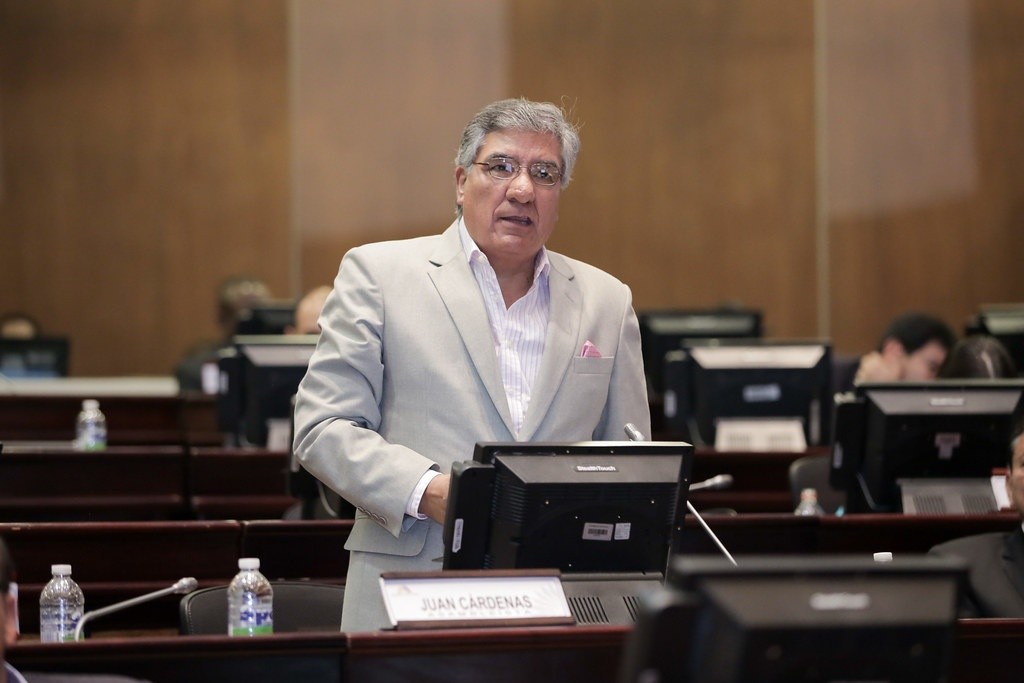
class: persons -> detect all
[835,311,1016,483]
[925,395,1024,618]
[291,98,653,632]
[0,310,38,339]
[239,283,335,451]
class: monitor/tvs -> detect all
[0,335,70,381]
[213,299,1023,683]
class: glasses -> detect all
[471,156,563,186]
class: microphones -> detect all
[75,577,199,642]
[620,422,738,565]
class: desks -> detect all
[0,379,1024,683]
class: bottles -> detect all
[792,488,826,516]
[227,558,273,636]
[39,564,85,641]
[76,399,108,449]
